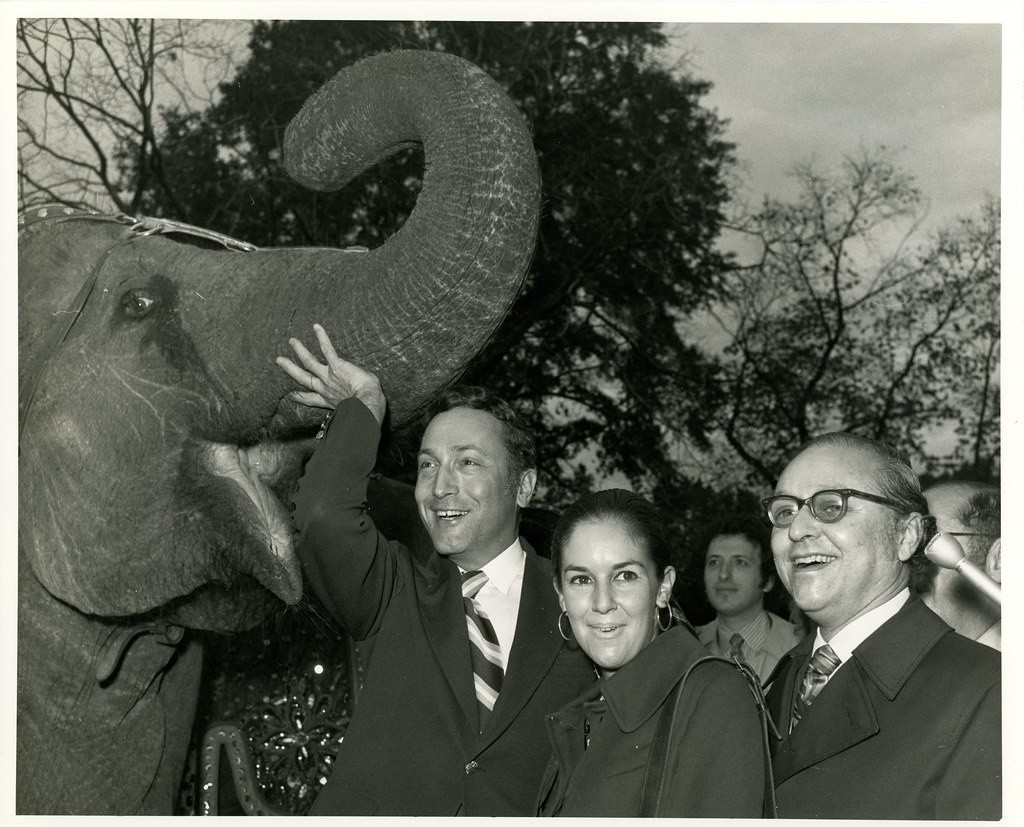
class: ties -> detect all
[788,643,842,736]
[462,569,504,732]
[729,633,745,663]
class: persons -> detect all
[274,323,603,816]
[673,512,808,689]
[920,478,1000,654]
[758,431,1002,820]
[532,488,783,818]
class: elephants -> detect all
[17,45,544,816]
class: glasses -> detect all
[762,486,912,529]
